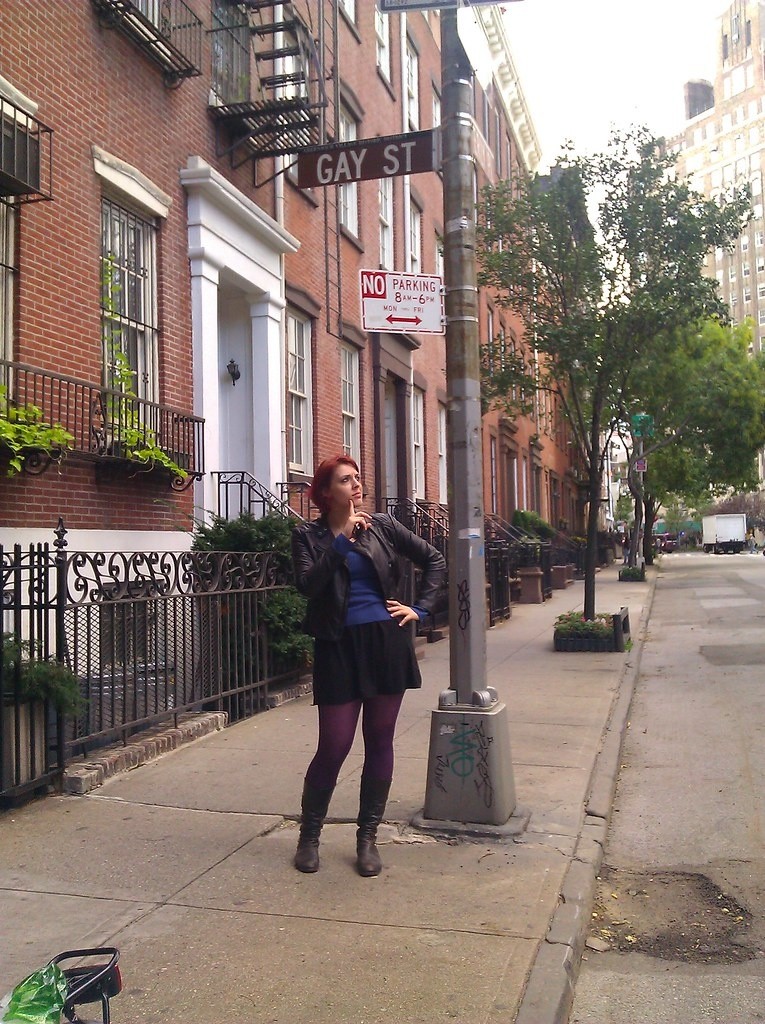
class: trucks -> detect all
[702,514,748,555]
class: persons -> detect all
[657,537,661,554]
[290,453,448,879]
[749,533,759,554]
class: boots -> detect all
[356,775,391,876]
[295,778,336,872]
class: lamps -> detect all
[227,358,241,387]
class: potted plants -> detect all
[3,631,91,801]
